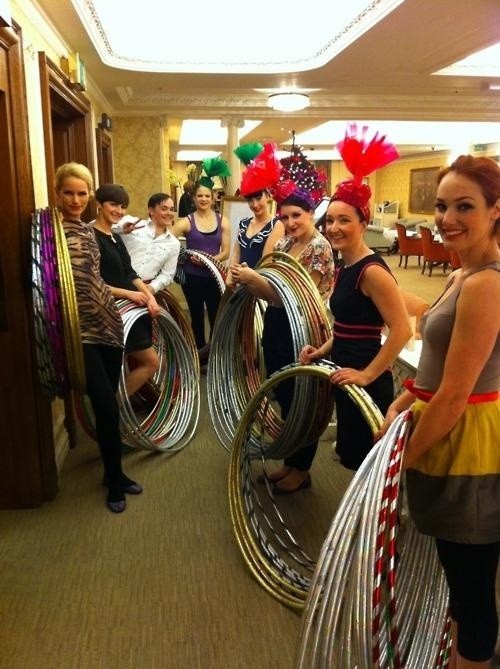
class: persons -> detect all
[230,194,336,496]
[179,180,196,217]
[111,193,181,294]
[55,162,143,514]
[225,188,285,292]
[373,155,500,669]
[92,184,160,409]
[300,193,413,470]
[170,181,231,374]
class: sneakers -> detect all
[103,477,142,512]
[256,469,311,495]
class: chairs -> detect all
[363,217,462,278]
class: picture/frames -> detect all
[408,166,444,215]
